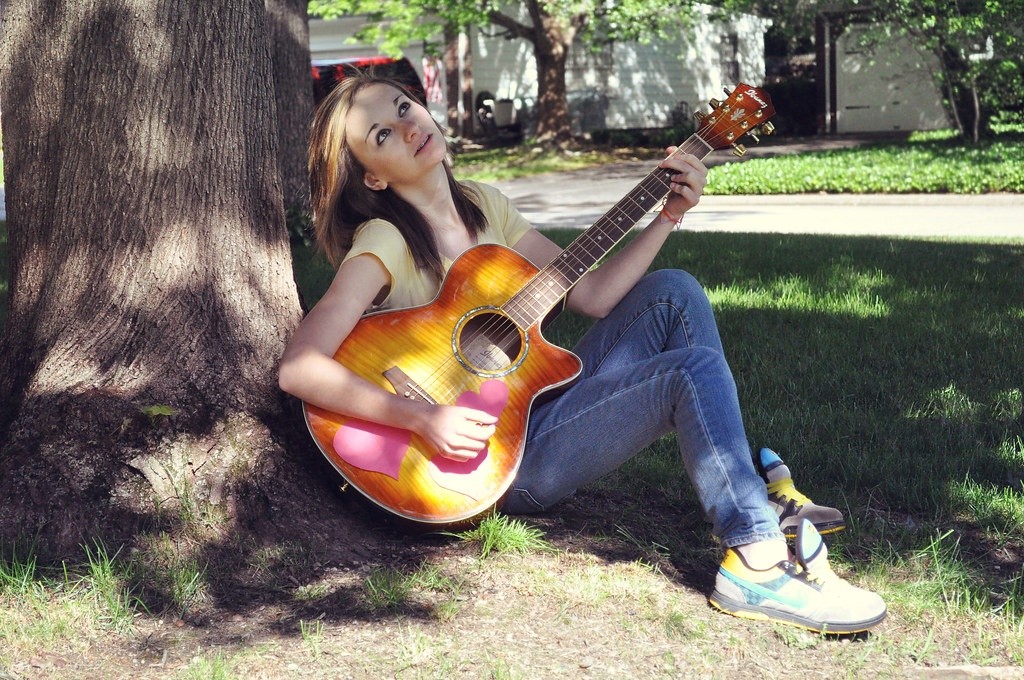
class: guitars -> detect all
[275,76,780,534]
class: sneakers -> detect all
[709,518,888,634]
[708,449,846,542]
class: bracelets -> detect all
[651,196,686,232]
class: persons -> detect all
[278,74,890,634]
